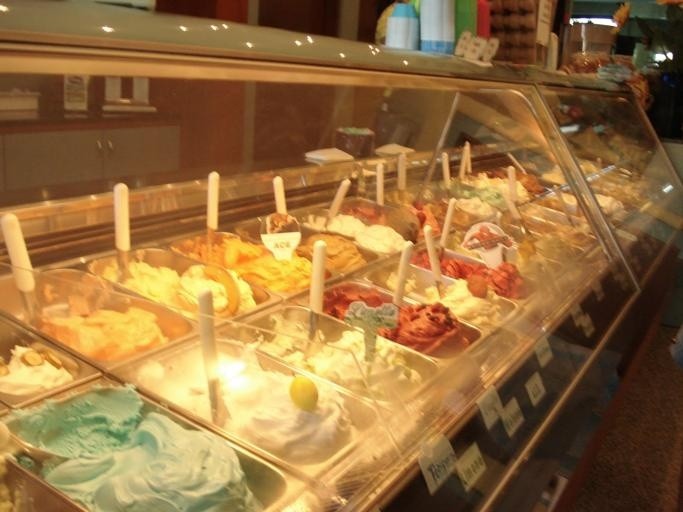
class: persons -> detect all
[357,0,414,159]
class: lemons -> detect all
[288,375,318,410]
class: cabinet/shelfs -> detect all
[0,0,683,512]
